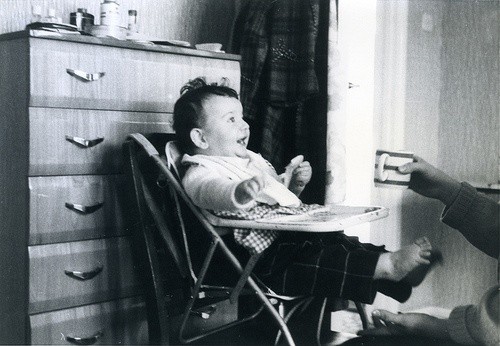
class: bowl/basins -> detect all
[195,43,222,51]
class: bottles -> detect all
[101,0,120,25]
[71,8,94,36]
[127,10,138,34]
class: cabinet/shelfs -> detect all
[0,29,241,345]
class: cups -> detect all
[373,150,414,193]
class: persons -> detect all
[170,77,431,306]
[338,150,500,346]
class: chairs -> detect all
[123,133,389,346]
